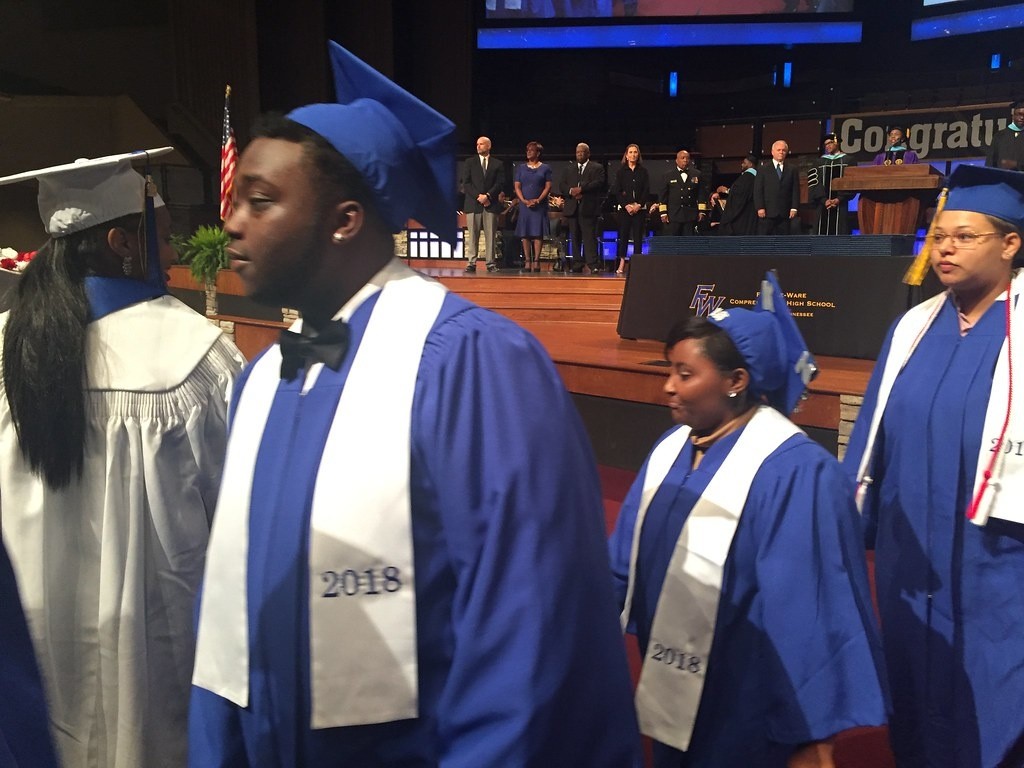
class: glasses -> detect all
[925,231,1008,248]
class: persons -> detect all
[607,272,889,767]
[874,126,919,164]
[808,133,858,235]
[186,41,645,768]
[615,144,649,274]
[463,137,506,272]
[985,99,1024,170]
[514,142,553,272]
[843,165,1024,768]
[0,146,250,768]
[660,151,707,236]
[708,153,760,235]
[754,140,800,235]
[559,143,606,273]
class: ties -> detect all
[776,163,782,180]
[578,165,583,187]
[482,158,487,177]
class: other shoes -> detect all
[465,265,476,272]
[591,267,599,273]
[487,265,499,272]
[570,268,582,273]
[616,270,623,273]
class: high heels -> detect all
[534,260,540,272]
[524,260,531,272]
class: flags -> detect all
[221,99,240,224]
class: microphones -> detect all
[886,138,901,160]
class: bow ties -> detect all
[679,170,688,174]
[279,317,350,381]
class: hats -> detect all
[706,270,817,418]
[888,125,907,144]
[822,133,843,145]
[901,164,1024,287]
[285,40,458,250]
[0,146,174,293]
[1008,99,1024,109]
[746,151,762,165]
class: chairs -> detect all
[595,213,647,271]
